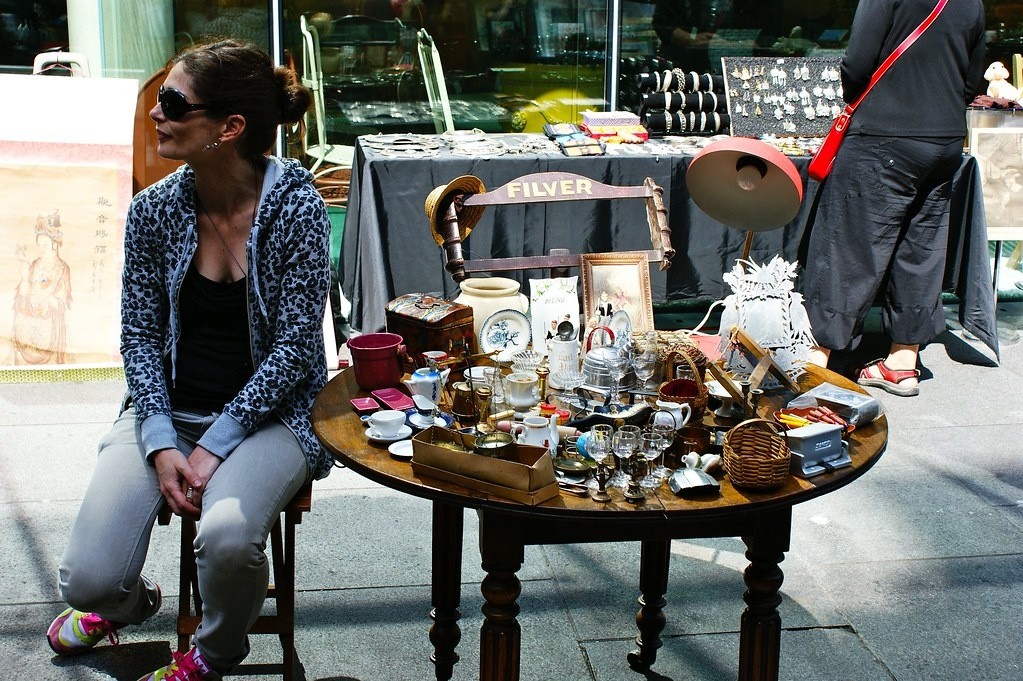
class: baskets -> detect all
[723,418,791,487]
[658,350,709,422]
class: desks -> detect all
[337,136,974,336]
[312,329,888,680]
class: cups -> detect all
[369,409,406,438]
[484,338,582,404]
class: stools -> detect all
[175,485,313,681]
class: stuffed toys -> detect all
[984,62,1019,100]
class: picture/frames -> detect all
[581,251,655,335]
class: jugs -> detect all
[652,396,692,438]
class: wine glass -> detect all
[550,369,585,404]
[607,347,656,406]
[587,423,674,490]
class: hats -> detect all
[425,175,486,248]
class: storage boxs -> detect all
[410,426,559,505]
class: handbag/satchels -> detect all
[807,112,851,181]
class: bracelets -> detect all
[676,110,687,132]
[663,92,671,110]
[703,72,714,91]
[707,91,718,112]
[689,70,700,92]
[712,111,721,132]
[663,111,673,132]
[675,91,686,110]
[689,111,696,132]
[653,70,661,92]
[671,67,685,91]
[660,69,672,92]
[701,111,706,132]
[697,90,703,112]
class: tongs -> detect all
[558,481,589,496]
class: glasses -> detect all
[155,84,210,122]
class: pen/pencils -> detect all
[807,406,849,430]
[780,413,813,427]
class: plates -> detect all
[464,365,499,384]
[387,440,413,457]
[366,425,413,442]
[553,457,590,472]
[478,309,532,362]
[403,409,454,431]
[605,310,633,353]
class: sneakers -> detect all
[46,581,162,655]
[137,645,222,681]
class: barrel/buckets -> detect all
[349,332,405,391]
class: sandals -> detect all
[855,357,920,396]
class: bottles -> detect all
[504,372,540,413]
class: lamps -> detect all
[685,136,804,273]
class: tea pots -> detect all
[405,367,451,408]
[511,413,560,465]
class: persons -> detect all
[544,314,571,340]
[793,0,986,395]
[392,0,524,99]
[42,40,333,681]
[651,1,759,74]
